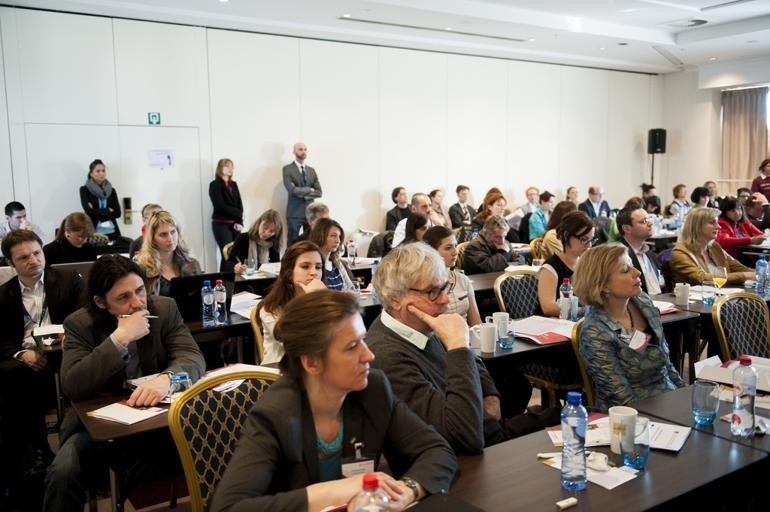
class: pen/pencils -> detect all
[236,256,242,264]
[118,315,158,318]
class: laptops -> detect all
[170,272,236,322]
[50,261,95,276]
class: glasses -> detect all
[407,281,453,303]
[579,237,598,245]
[639,217,649,224]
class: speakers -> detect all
[648,128,665,153]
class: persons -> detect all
[39,252,209,511]
[282,142,323,246]
[208,288,459,512]
[1,158,769,459]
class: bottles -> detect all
[351,474,390,512]
[346,239,356,270]
[764,261,770,300]
[729,354,758,437]
[559,392,590,495]
[651,209,682,235]
[370,260,380,302]
[755,255,768,294]
[559,278,574,320]
[472,229,478,240]
[201,278,226,327]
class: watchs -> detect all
[398,476,419,503]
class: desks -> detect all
[382,381,770,511]
[71,359,287,512]
[29,244,531,446]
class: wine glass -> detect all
[713,266,728,298]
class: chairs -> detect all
[710,292,769,366]
[167,369,284,511]
[491,265,580,406]
[570,316,593,412]
[664,204,672,215]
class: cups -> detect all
[242,258,255,276]
[618,415,650,468]
[672,281,715,306]
[485,312,509,336]
[169,371,192,408]
[346,280,360,292]
[690,379,721,427]
[498,318,516,350]
[452,258,545,274]
[570,298,585,321]
[609,405,637,456]
[338,243,345,257]
[471,323,497,353]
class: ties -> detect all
[463,207,469,220]
[301,166,305,182]
